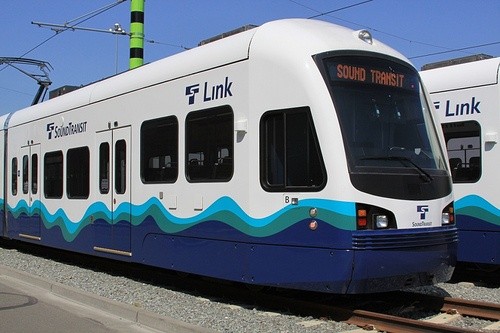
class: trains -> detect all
[417,53,500,274]
[0,17,460,298]
